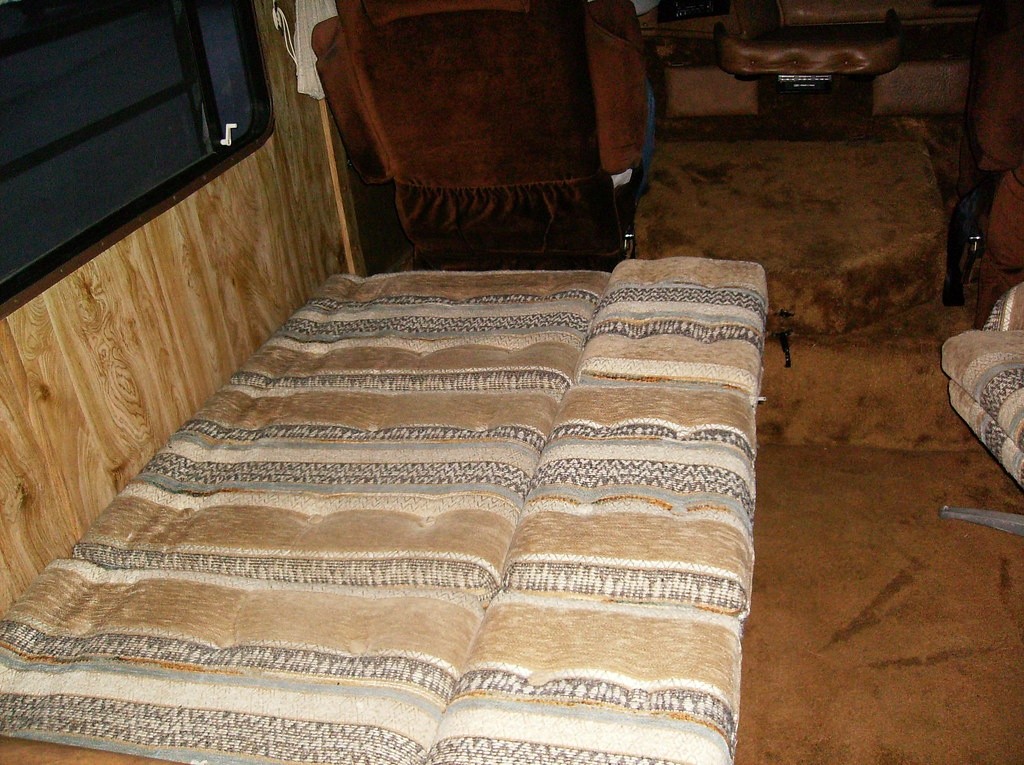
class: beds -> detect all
[0,257,769,764]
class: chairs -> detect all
[311,1,652,270]
[949,0,1024,331]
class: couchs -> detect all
[941,280,1024,490]
